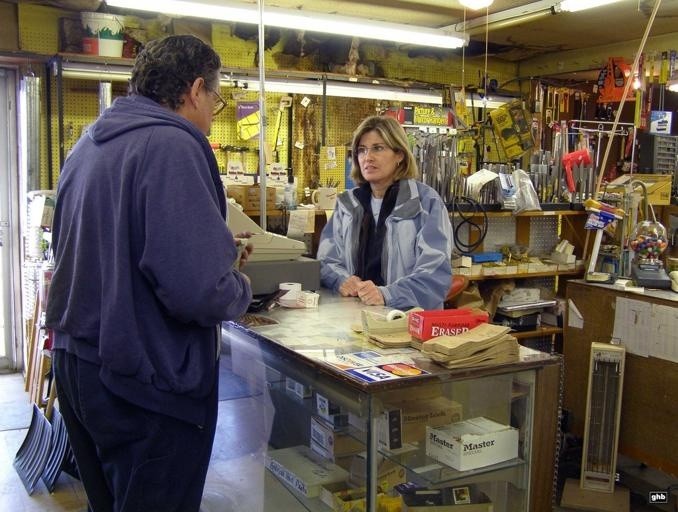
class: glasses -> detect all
[356,143,389,157]
[191,70,228,117]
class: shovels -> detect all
[14,349,70,497]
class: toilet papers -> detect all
[284,166,296,211]
[279,282,301,300]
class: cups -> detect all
[311,187,338,210]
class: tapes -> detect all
[279,282,301,292]
[326,176,340,188]
[387,309,405,323]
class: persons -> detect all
[40,34,253,509]
[315,113,454,313]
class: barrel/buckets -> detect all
[79,10,125,58]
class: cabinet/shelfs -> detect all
[449,210,589,339]
[199,327,535,512]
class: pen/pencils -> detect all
[267,302,276,311]
